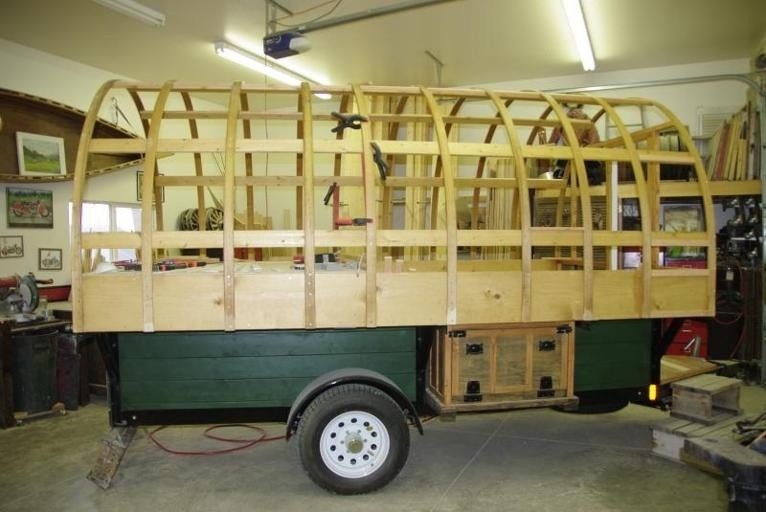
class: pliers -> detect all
[371,142,388,180]
[335,218,374,226]
[324,182,337,204]
[331,112,368,132]
[116,259,207,272]
[292,253,341,269]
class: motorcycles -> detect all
[11,196,51,219]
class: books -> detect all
[703,104,762,182]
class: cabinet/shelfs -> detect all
[660,257,764,364]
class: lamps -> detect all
[93,0,169,33]
[559,1,601,76]
[208,39,333,103]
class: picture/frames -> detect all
[37,247,63,272]
[13,127,67,178]
[135,170,165,204]
[0,235,25,258]
